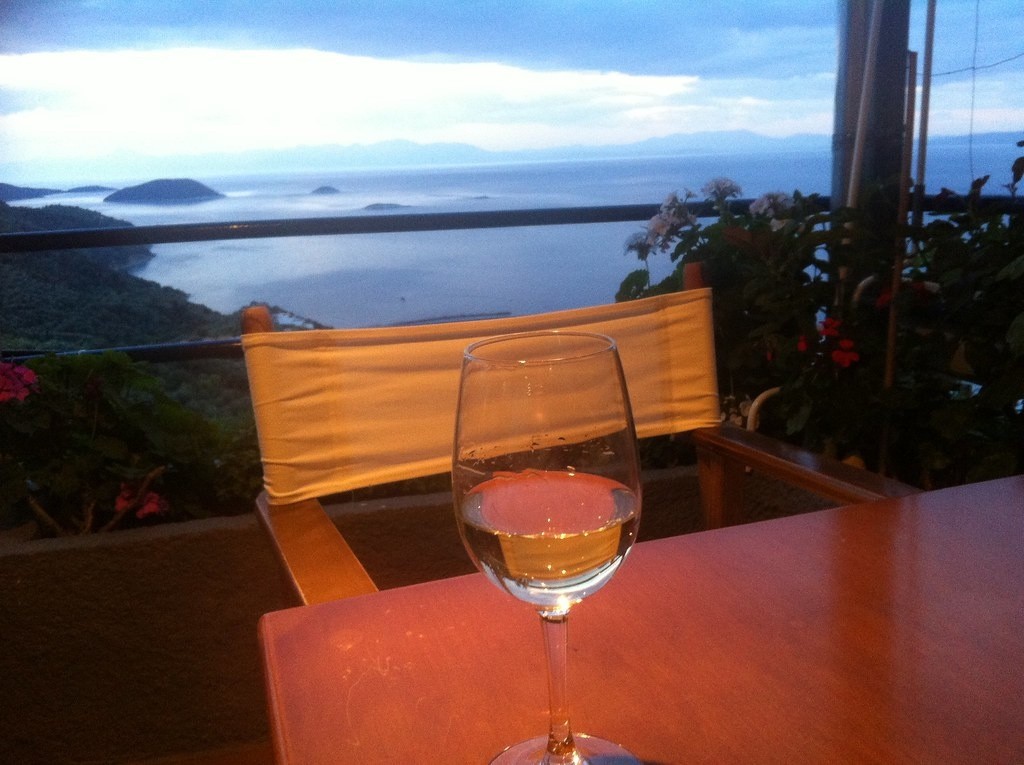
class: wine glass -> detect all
[452,331,643,765]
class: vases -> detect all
[628,467,845,545]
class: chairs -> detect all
[238,260,924,606]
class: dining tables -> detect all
[257,474,1024,765]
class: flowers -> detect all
[116,482,160,518]
[613,137,1024,494]
[0,361,37,402]
